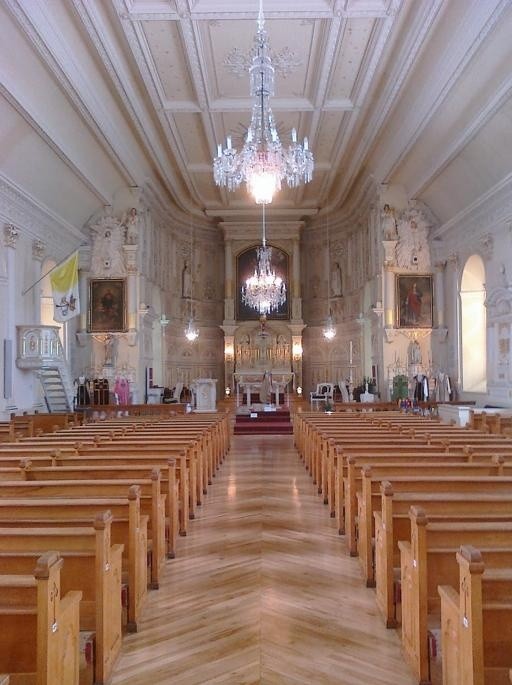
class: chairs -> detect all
[309,382,336,406]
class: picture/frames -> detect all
[87,276,129,334]
[394,272,436,331]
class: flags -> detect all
[49,248,83,324]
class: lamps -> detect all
[211,0,316,315]
[321,195,338,340]
[183,195,200,341]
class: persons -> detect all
[97,332,115,366]
[404,280,423,324]
[411,338,423,364]
[278,336,286,357]
[183,259,194,298]
[380,202,397,241]
[122,206,139,244]
[240,335,250,357]
[98,287,118,319]
[329,261,342,298]
